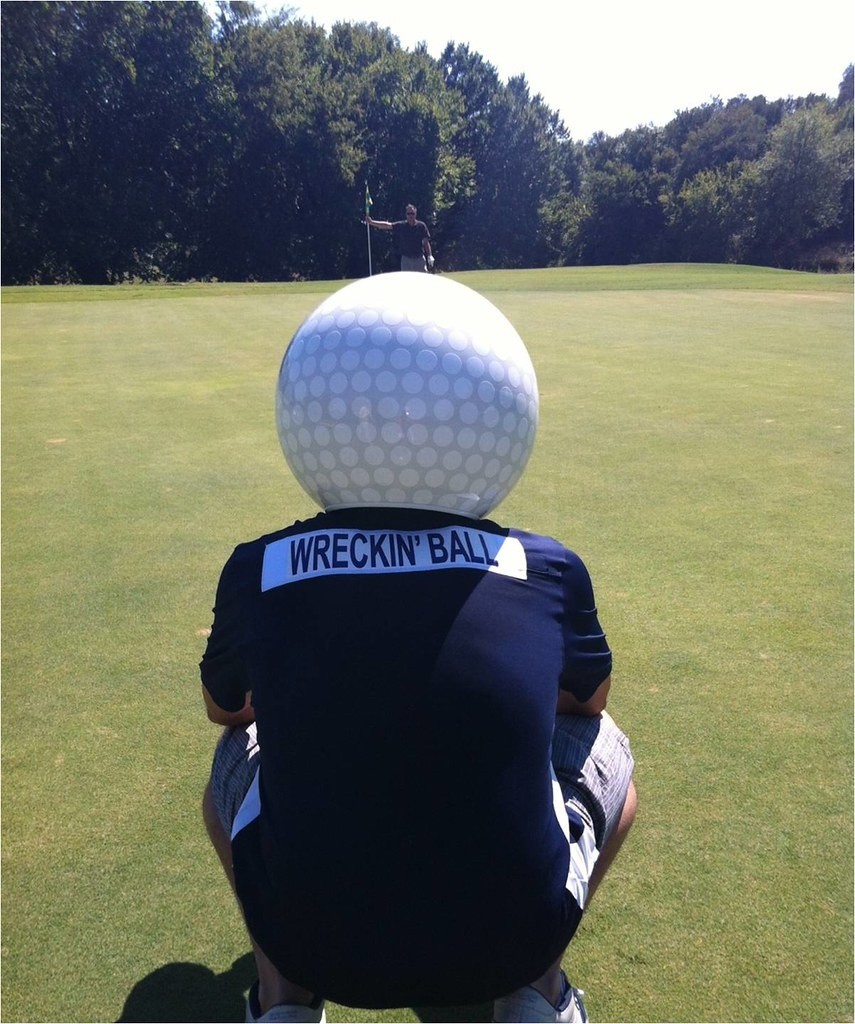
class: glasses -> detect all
[407,212,416,216]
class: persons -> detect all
[366,204,435,273]
[199,508,639,1023]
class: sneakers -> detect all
[245,984,327,1023]
[492,967,589,1023]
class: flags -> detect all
[366,187,373,208]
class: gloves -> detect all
[428,255,435,268]
